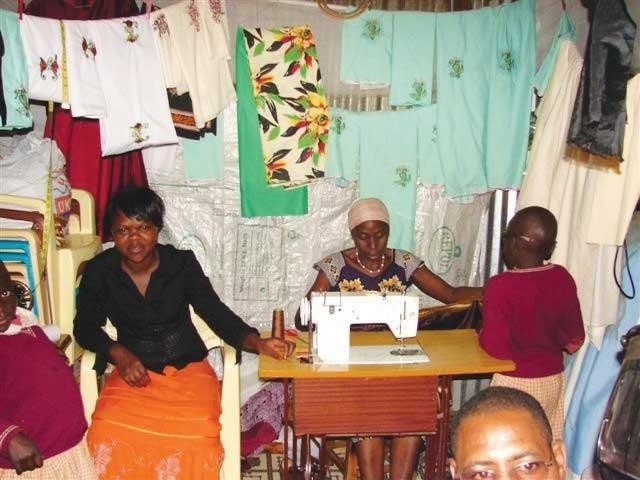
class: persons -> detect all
[476,206,586,475]
[447,385,564,479]
[72,186,296,480]
[295,197,485,480]
[1,260,99,480]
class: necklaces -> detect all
[353,247,385,275]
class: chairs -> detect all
[79,304,240,479]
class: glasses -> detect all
[454,452,561,480]
